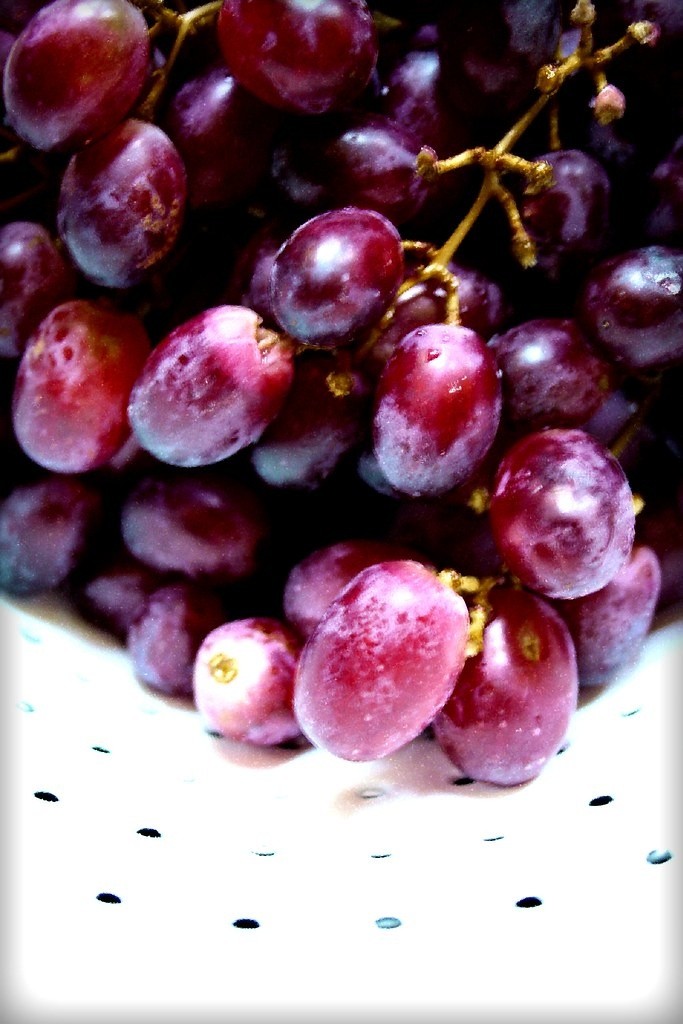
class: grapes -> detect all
[0,0,683,787]
